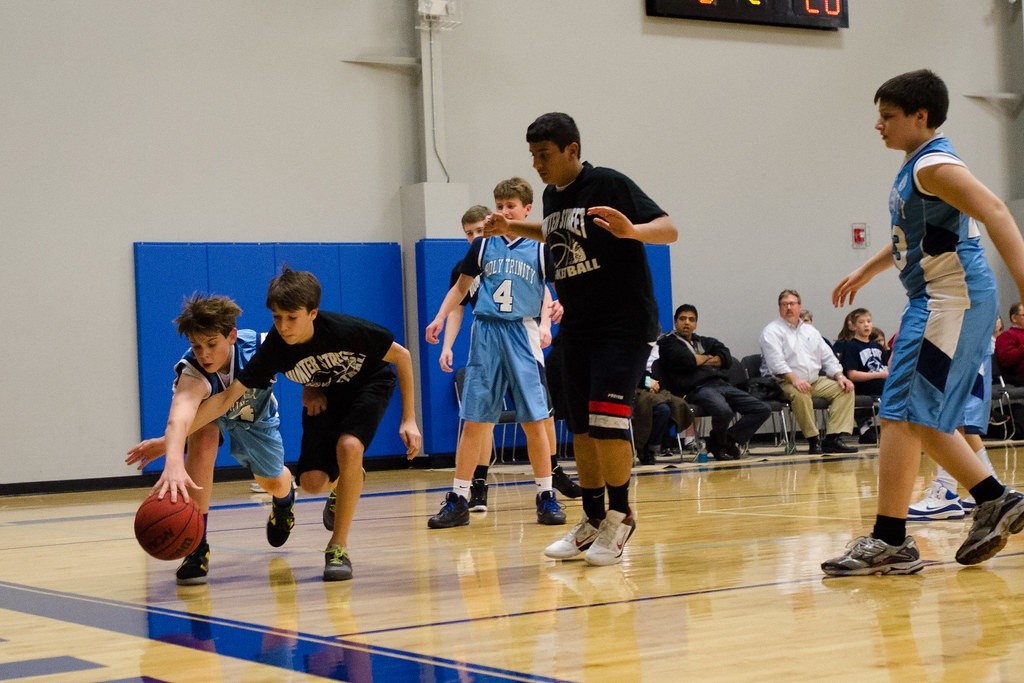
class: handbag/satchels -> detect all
[736,377,784,402]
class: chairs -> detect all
[980,376,1024,442]
[456,367,580,465]
[629,355,880,465]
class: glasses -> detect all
[780,301,799,307]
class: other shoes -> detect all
[684,437,706,452]
[637,449,655,464]
[657,447,679,457]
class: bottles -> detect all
[696,436,708,462]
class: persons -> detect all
[148,291,326,579]
[439,203,582,512]
[124,268,421,581]
[821,71,1024,574]
[991,314,1003,351]
[423,176,566,530]
[996,301,1024,387]
[484,112,677,564]
[632,290,897,466]
[906,340,1000,520]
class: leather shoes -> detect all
[705,437,741,459]
[821,437,858,453]
[809,439,823,454]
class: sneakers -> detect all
[468,479,489,511]
[545,512,601,559]
[323,467,366,531]
[266,481,296,547]
[955,484,1024,565]
[536,490,567,525]
[176,542,211,584]
[821,535,924,576]
[584,506,637,565]
[323,545,352,580]
[552,465,582,498]
[907,480,976,520]
[427,491,469,528]
[251,475,298,491]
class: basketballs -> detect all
[134,491,204,561]
